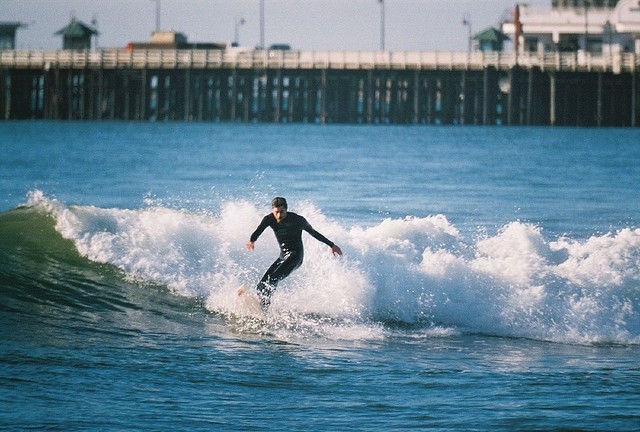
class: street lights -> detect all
[233,17,246,50]
[461,11,474,51]
[90,17,99,49]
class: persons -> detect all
[247,197,342,311]
